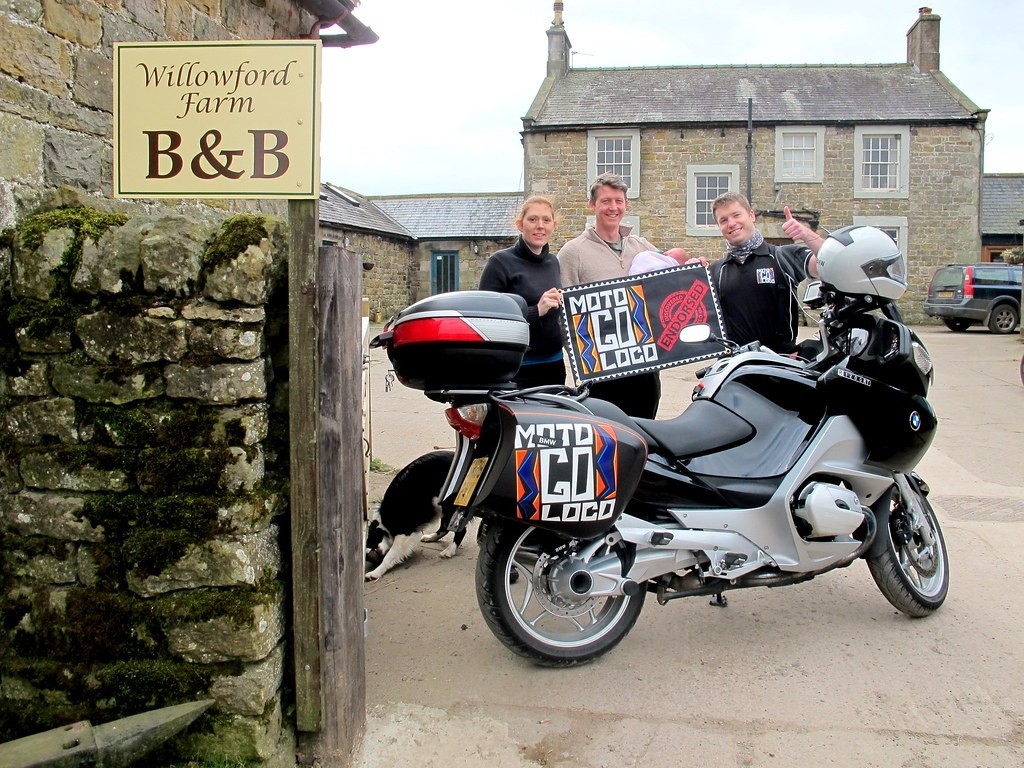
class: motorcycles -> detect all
[369,280,948,665]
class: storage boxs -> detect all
[471,389,648,539]
[368,291,530,390]
[436,430,474,508]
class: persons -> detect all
[556,174,709,422]
[480,198,566,389]
[628,248,689,277]
[706,192,825,361]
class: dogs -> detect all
[366,450,475,581]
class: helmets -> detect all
[816,226,907,302]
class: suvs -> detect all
[923,261,1024,334]
